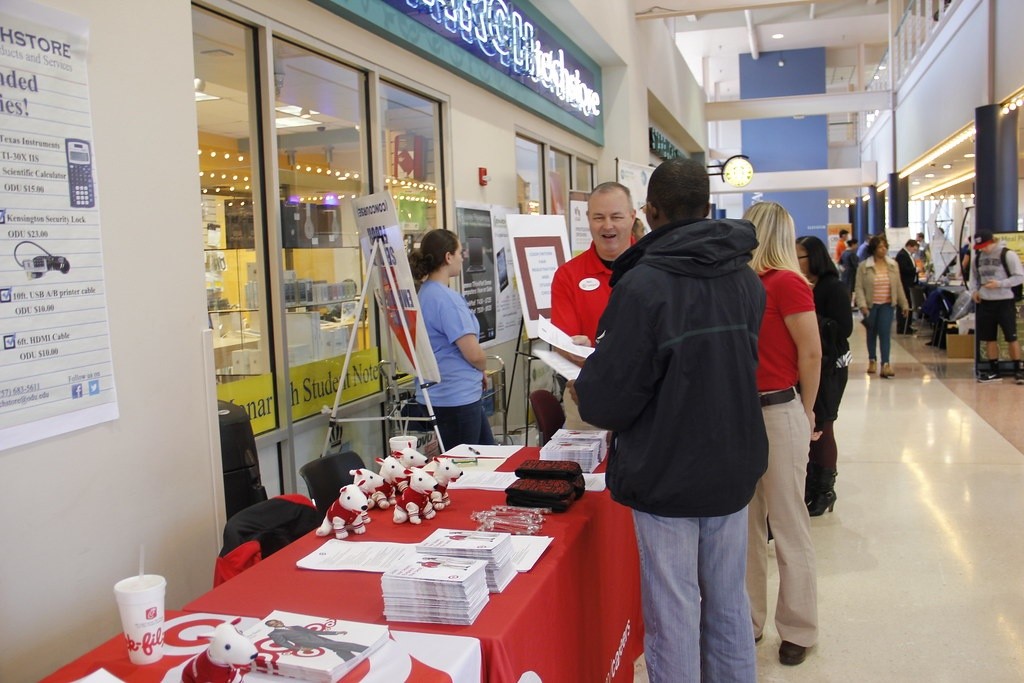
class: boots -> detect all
[805,463,837,516]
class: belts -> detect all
[759,384,801,407]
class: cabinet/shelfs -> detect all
[199,191,366,376]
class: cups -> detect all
[389,435,418,454]
[113,575,167,665]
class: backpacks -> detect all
[976,247,1023,302]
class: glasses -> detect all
[639,204,647,213]
[797,255,809,258]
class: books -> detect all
[237,528,518,683]
[539,428,609,473]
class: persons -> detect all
[738,201,825,664]
[409,228,496,454]
[550,181,1024,542]
[265,620,368,662]
[416,562,470,571]
[566,161,769,683]
[421,558,479,566]
[449,532,501,538]
[443,535,495,543]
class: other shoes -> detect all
[1016,369,1024,384]
[881,363,895,376]
[867,357,878,373]
[779,640,807,665]
[978,372,1003,382]
[897,327,912,334]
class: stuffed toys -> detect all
[314,441,464,540]
[159,618,258,683]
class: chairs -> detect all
[212,452,367,589]
[905,282,955,351]
[530,389,566,446]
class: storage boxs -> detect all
[946,324,974,359]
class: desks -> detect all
[911,279,969,321]
[39,447,647,683]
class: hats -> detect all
[973,230,993,250]
